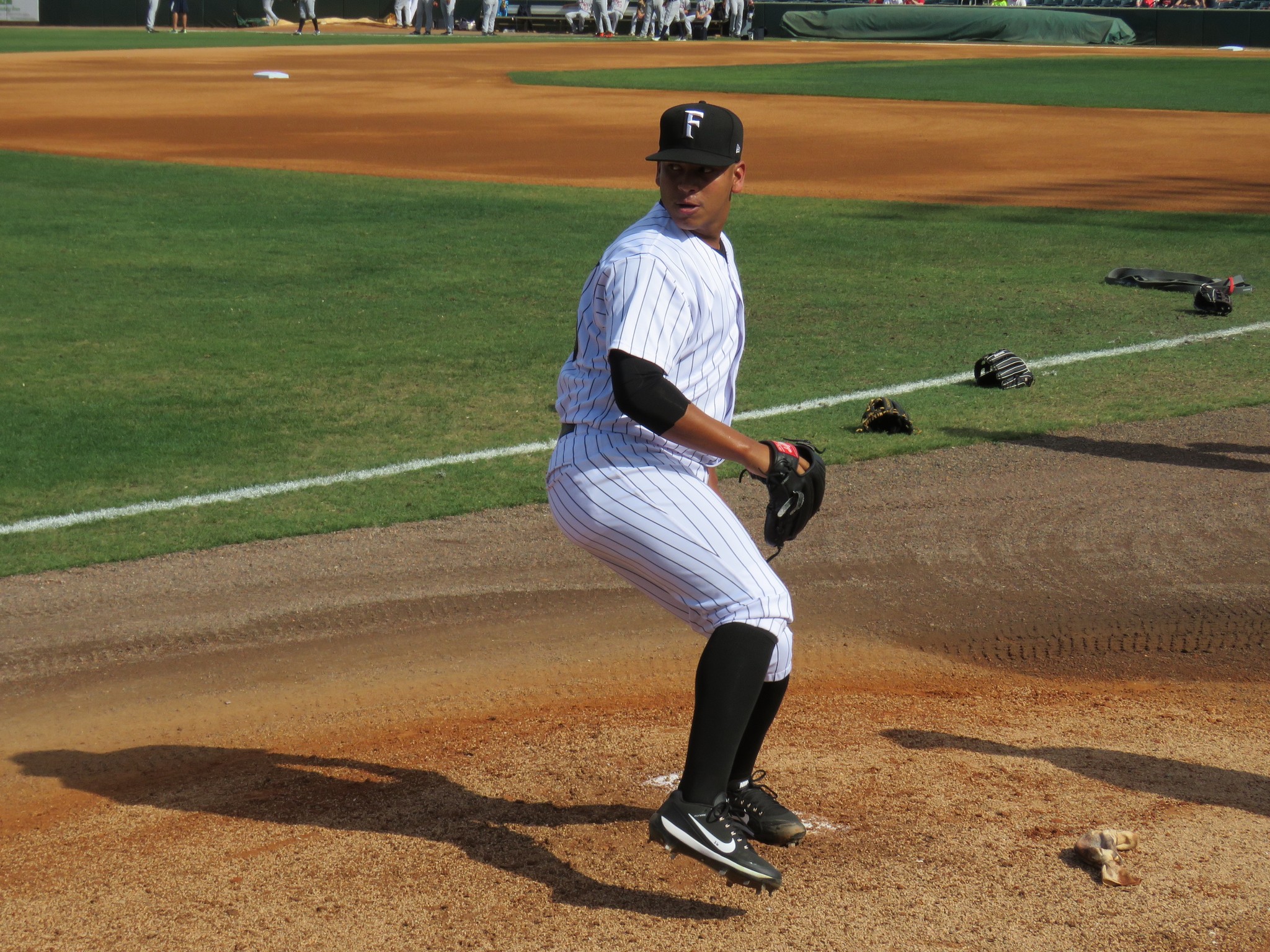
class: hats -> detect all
[645,101,744,166]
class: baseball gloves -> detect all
[974,348,1034,389]
[858,396,914,436]
[753,440,827,547]
[1194,283,1233,315]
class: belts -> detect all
[558,423,576,437]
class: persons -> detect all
[869,0,1209,9]
[293,0,319,36]
[262,0,280,26]
[547,99,827,895]
[168,0,190,34]
[145,0,160,32]
[393,0,756,44]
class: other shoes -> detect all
[180,29,186,33]
[393,20,496,38]
[148,28,159,33]
[566,21,755,44]
[273,18,279,26]
[291,31,301,36]
[169,30,178,34]
[313,31,320,35]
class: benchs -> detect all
[480,5,729,36]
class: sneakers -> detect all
[648,791,782,897]
[724,770,808,846]
